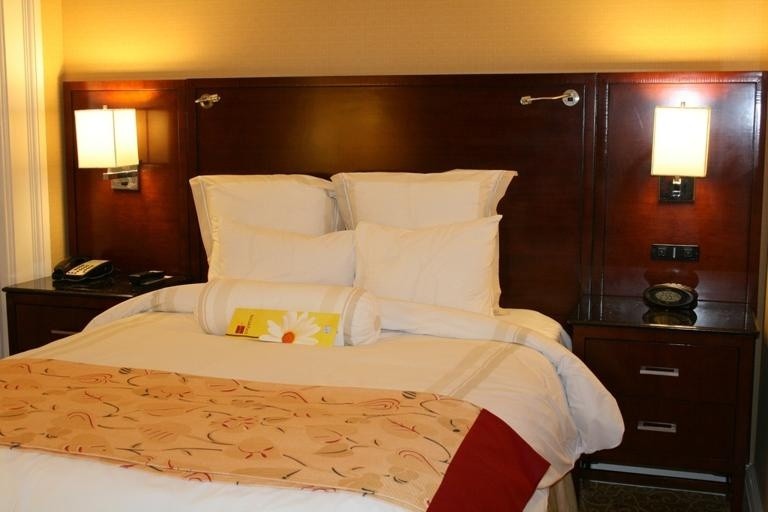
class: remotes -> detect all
[129,269,165,284]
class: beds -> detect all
[2,274,631,510]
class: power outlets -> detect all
[649,244,698,262]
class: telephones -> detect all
[52,256,115,284]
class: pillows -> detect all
[205,215,358,287]
[329,167,519,232]
[187,172,345,266]
[352,214,504,320]
[195,277,382,349]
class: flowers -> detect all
[258,310,321,348]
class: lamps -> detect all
[520,88,582,107]
[71,103,145,198]
[130,106,175,172]
[647,99,713,207]
[195,92,221,109]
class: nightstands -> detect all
[1,273,200,356]
[566,291,762,511]
